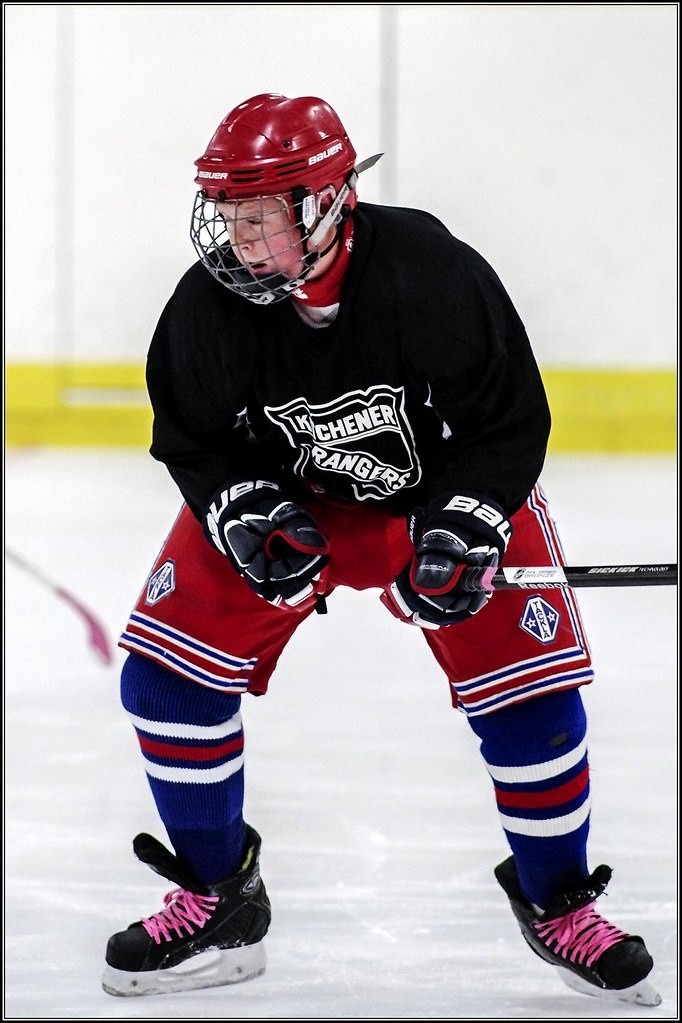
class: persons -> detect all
[101,93,663,1007]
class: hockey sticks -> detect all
[452,564,676,592]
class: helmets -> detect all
[189,92,357,306]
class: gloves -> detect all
[202,476,331,612]
[380,492,514,630]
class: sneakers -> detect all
[101,823,273,998]
[494,853,662,1006]
